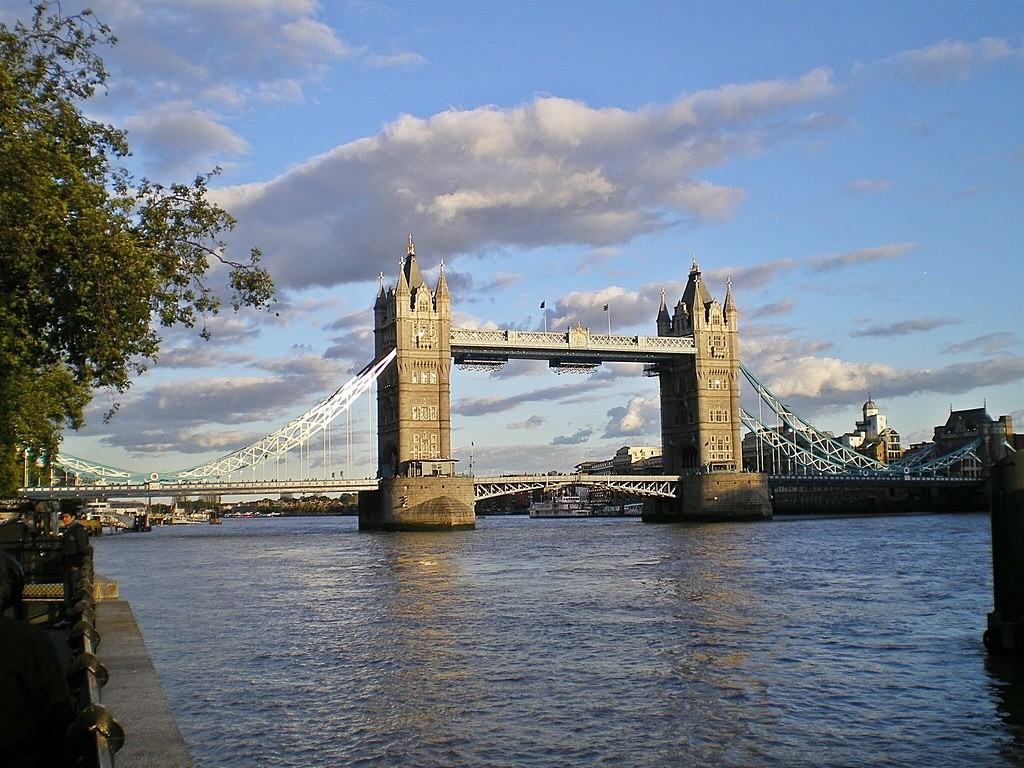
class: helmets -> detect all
[0,551,24,610]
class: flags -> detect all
[539,300,546,308]
[603,303,608,310]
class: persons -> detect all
[52,507,88,630]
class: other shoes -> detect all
[48,609,59,625]
[49,617,71,628]
